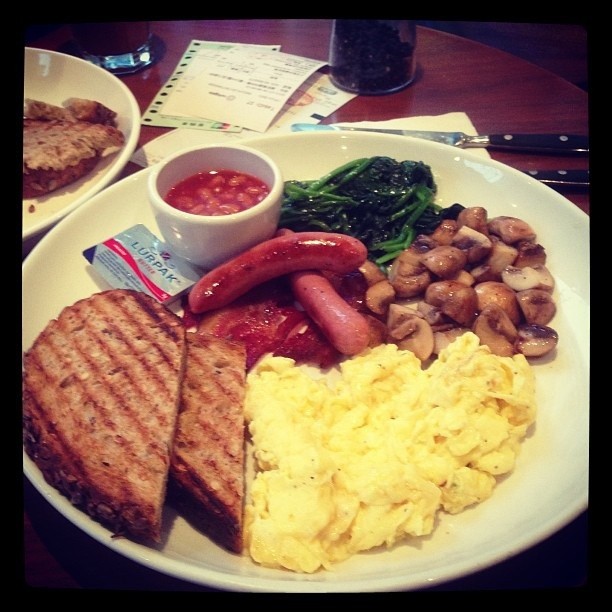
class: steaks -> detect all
[22,97,126,201]
[23,288,247,557]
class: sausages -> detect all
[187,233,368,312]
[278,227,371,356]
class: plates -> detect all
[24,46,140,237]
[23,130,588,595]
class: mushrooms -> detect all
[365,206,559,360]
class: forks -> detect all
[512,168,588,195]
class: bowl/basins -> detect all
[147,143,284,268]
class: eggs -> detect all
[244,332,538,574]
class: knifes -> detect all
[290,123,590,157]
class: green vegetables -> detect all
[279,156,467,270]
